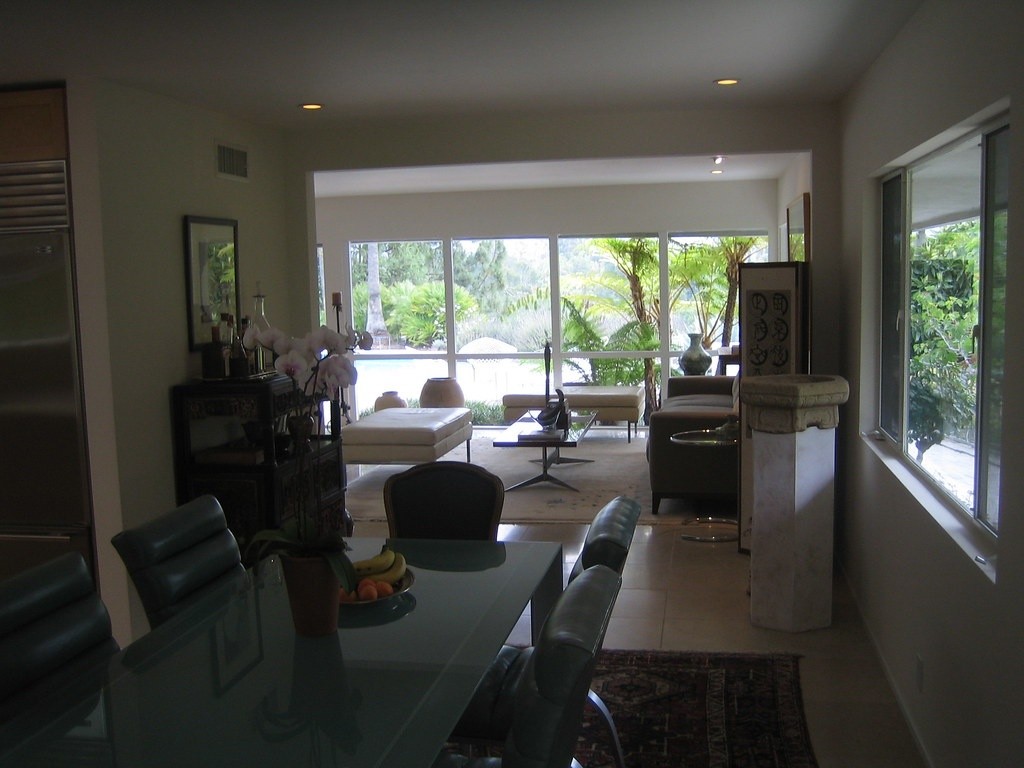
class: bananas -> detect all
[352,546,407,585]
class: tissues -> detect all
[548,389,572,431]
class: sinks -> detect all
[672,431,732,443]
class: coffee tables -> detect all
[493,407,599,493]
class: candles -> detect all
[331,292,341,306]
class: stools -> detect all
[341,408,473,467]
[502,385,645,444]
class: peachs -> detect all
[338,579,393,602]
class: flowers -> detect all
[241,325,373,596]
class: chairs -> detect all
[436,565,622,768]
[384,460,505,543]
[112,493,246,631]
[1,553,123,720]
[442,496,642,768]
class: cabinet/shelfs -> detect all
[172,380,350,541]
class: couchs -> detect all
[645,375,740,516]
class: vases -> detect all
[280,552,339,636]
[679,334,712,376]
[288,414,315,451]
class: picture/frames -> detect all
[212,579,265,700]
[184,214,241,350]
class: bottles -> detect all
[202,296,271,379]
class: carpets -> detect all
[345,429,738,531]
[439,643,821,768]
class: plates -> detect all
[341,567,416,605]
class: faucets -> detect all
[715,415,739,432]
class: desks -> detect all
[0,538,564,768]
